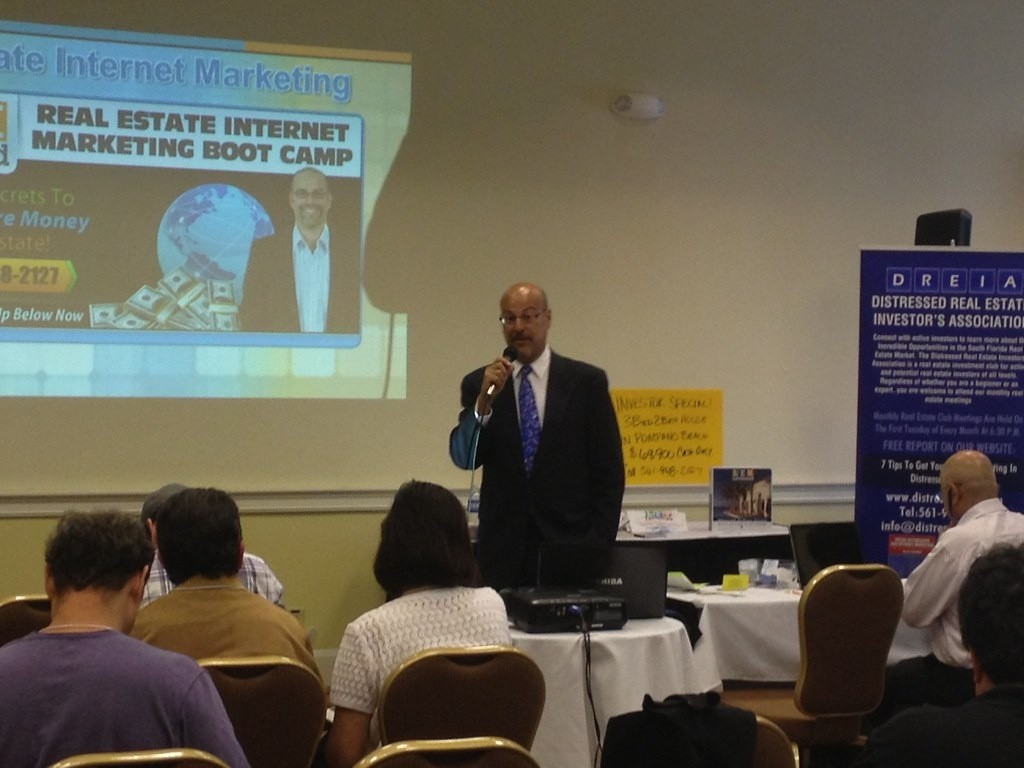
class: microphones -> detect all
[485,346,517,395]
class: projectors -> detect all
[500,586,628,635]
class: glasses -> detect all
[499,306,547,326]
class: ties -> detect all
[518,365,541,473]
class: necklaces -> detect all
[35,624,111,630]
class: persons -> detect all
[238,168,359,334]
[447,282,626,586]
[856,544,1024,768]
[0,508,252,768]
[134,482,287,612]
[860,449,1024,736]
[125,487,321,683]
[311,480,510,768]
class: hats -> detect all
[140,482,187,523]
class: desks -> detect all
[464,519,793,650]
[669,579,932,692]
[505,617,697,768]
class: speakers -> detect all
[914,208,971,247]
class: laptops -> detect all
[789,521,864,591]
[585,546,669,619]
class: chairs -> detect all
[0,523,1024,768]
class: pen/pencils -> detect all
[633,534,645,538]
[792,590,802,594]
